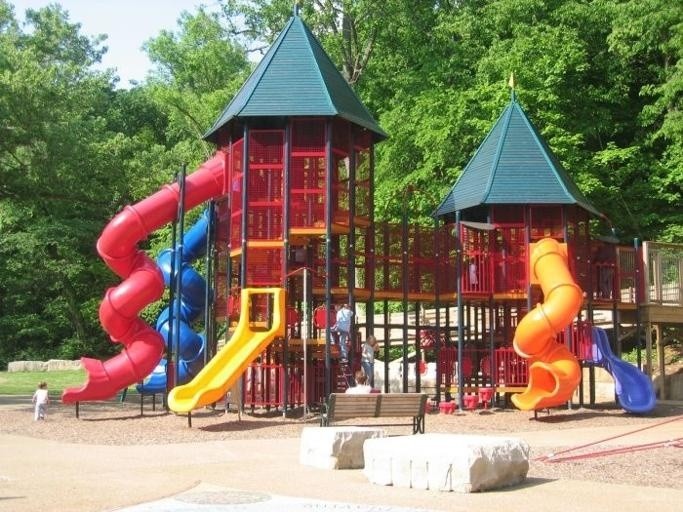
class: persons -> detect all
[31,381,51,422]
[344,370,373,393]
[360,335,379,385]
[330,304,354,364]
[469,257,479,291]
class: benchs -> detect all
[321,394,428,433]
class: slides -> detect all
[510,237,585,410]
[590,330,656,417]
[61,146,286,416]
